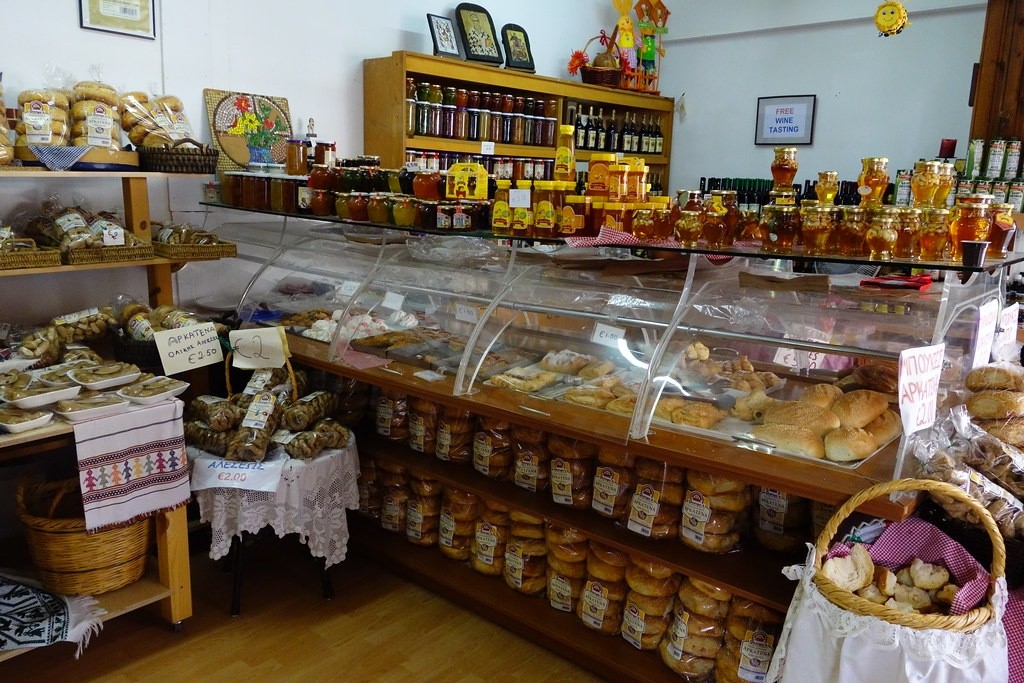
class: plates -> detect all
[0,359,189,433]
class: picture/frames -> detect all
[754,94,816,145]
[501,24,535,70]
[427,14,460,60]
[456,3,504,64]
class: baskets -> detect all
[15,469,150,596]
[52,229,154,265]
[138,138,219,173]
[0,237,62,270]
[578,35,625,87]
[815,477,1006,630]
[151,221,238,260]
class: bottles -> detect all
[700,178,895,206]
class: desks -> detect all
[186,430,356,617]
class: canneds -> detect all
[220,138,556,232]
[406,79,558,147]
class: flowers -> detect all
[567,48,631,75]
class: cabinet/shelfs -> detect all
[200,318,965,683]
[363,50,675,198]
[0,174,221,683]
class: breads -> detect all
[283,308,538,372]
[819,542,964,618]
[487,342,909,465]
[0,304,227,426]
[349,386,844,683]
[915,363,1024,543]
[0,81,198,166]
[0,206,219,256]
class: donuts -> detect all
[181,368,350,462]
